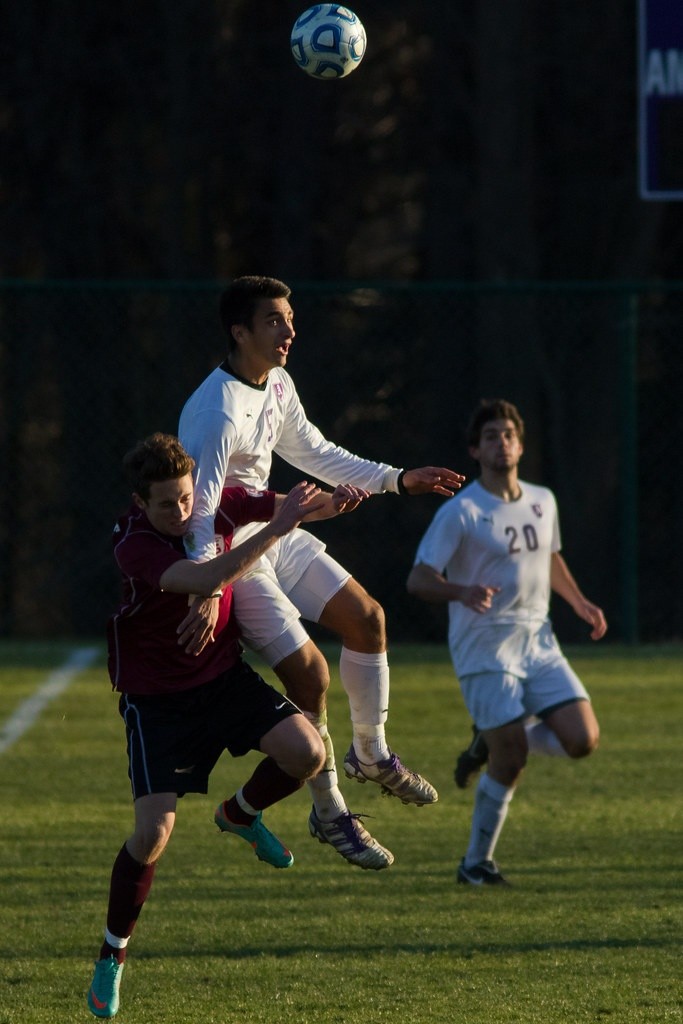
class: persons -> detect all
[406,401,607,889]
[85,433,371,1021]
[175,277,466,870]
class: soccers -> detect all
[289,2,366,80]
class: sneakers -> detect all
[455,724,492,789]
[343,743,439,807]
[308,802,395,870]
[88,953,123,1018]
[214,801,295,868]
[456,856,507,887]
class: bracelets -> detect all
[397,470,408,496]
